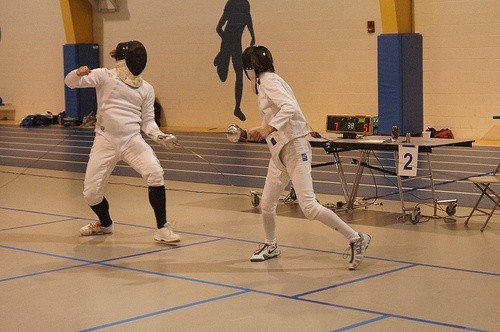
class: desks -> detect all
[306,132,478,222]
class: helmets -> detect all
[241,46,276,95]
[115,41,147,88]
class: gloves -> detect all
[162,136,177,152]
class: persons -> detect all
[64,41,181,244]
[241,46,371,270]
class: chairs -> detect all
[463,160,500,231]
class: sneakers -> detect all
[154,223,181,243]
[79,220,114,235]
[343,231,372,270]
[250,240,281,262]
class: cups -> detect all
[422,131,430,142]
[392,125,398,142]
